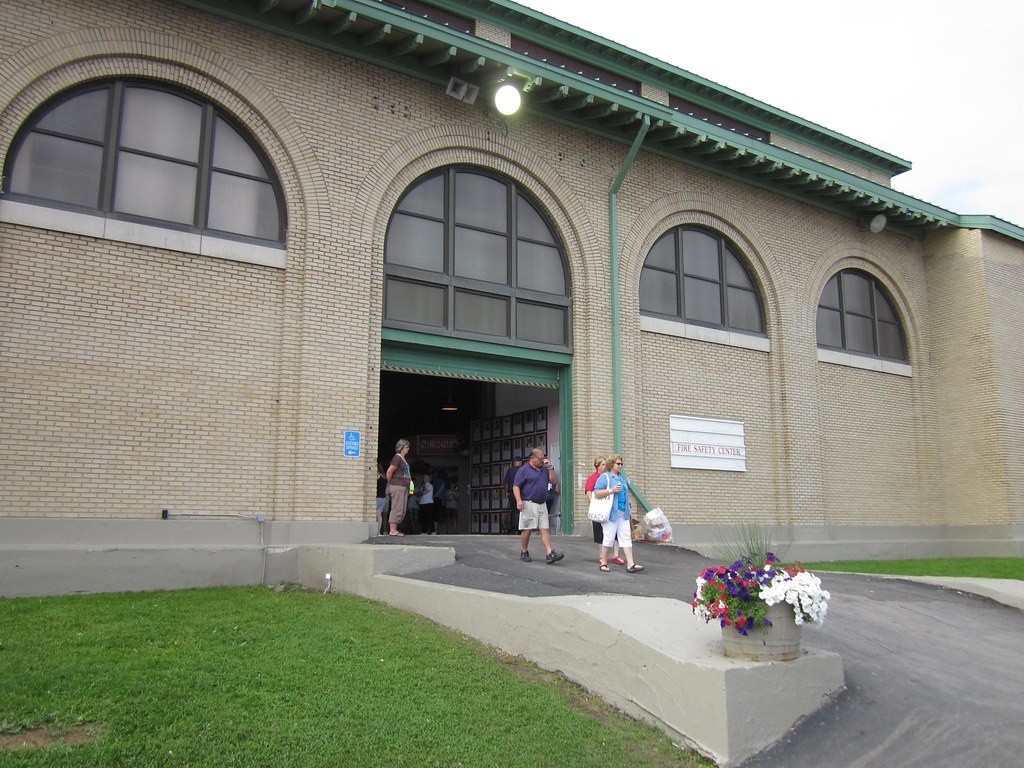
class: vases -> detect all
[720,598,802,663]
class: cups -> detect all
[617,483,622,491]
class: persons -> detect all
[385,439,411,537]
[504,456,523,535]
[512,448,564,564]
[407,474,459,535]
[585,456,625,564]
[594,453,645,572]
[377,463,389,534]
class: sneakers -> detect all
[546,549,564,563]
[521,550,531,562]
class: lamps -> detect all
[860,212,887,236]
[484,83,522,117]
[439,377,464,416]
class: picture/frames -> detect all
[471,444,481,465]
[480,442,491,464]
[500,439,512,461]
[523,435,535,460]
[523,410,535,434]
[535,433,547,456]
[512,437,523,461]
[481,417,492,440]
[490,441,501,462]
[470,488,511,535]
[535,407,548,432]
[512,412,523,436]
[471,420,483,443]
[501,415,512,438]
[491,416,501,439]
[491,464,501,486]
[470,467,480,488]
[480,466,491,487]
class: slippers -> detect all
[627,564,644,573]
[599,563,610,572]
[390,531,405,537]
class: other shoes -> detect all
[612,556,625,565]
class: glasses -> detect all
[536,456,543,462]
[614,462,623,466]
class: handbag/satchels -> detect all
[409,480,414,495]
[588,473,614,522]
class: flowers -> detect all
[688,517,832,637]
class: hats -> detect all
[513,456,522,461]
